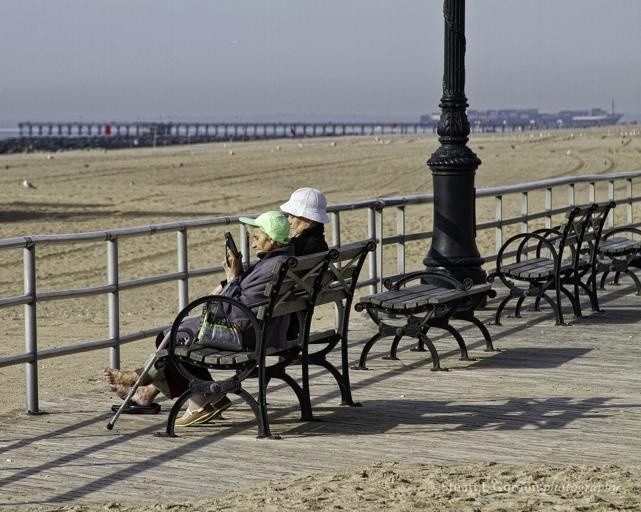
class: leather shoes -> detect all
[111,399,161,414]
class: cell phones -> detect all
[224,231,239,259]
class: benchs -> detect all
[486,201,616,326]
[155,239,379,440]
[574,226,641,296]
[351,272,497,371]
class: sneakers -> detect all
[174,394,232,426]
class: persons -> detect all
[105,188,331,412]
[153,211,296,426]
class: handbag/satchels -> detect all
[196,300,244,351]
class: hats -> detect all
[239,211,290,245]
[280,187,330,223]
